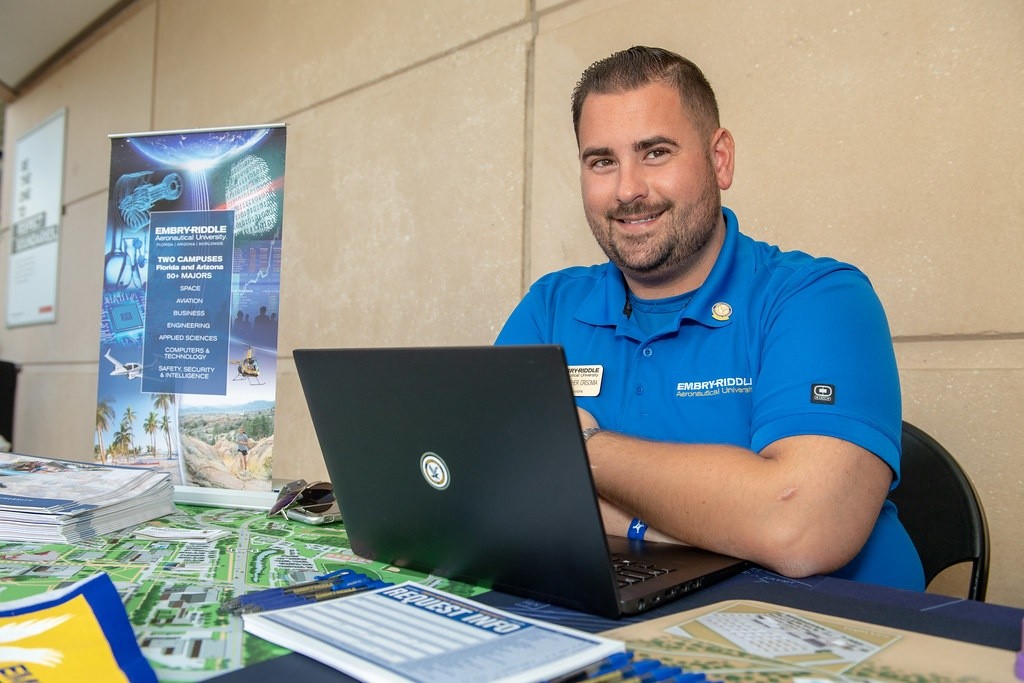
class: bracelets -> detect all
[627,518,648,540]
[582,427,603,443]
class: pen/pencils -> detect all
[541,653,724,683]
[216,567,394,612]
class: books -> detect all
[241,579,626,683]
[0,452,177,544]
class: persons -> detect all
[236,426,248,475]
[493,44,927,593]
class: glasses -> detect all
[266,481,334,521]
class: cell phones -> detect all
[287,498,343,526]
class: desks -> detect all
[1,505,1024,683]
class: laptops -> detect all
[293,344,755,620]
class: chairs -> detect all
[881,420,989,601]
